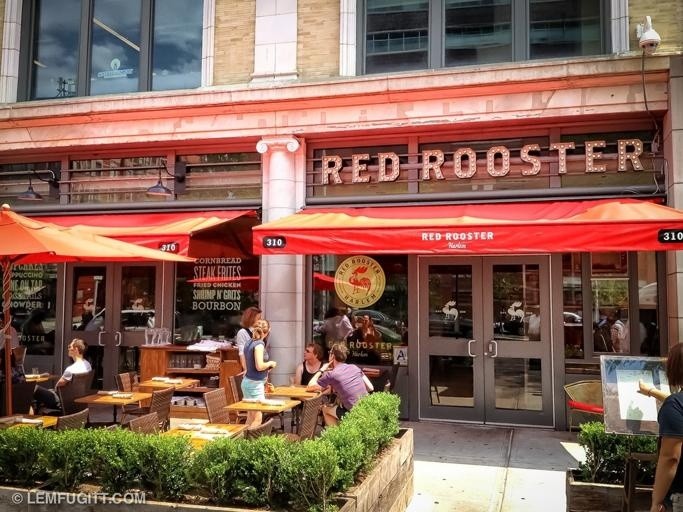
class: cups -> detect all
[169,353,203,369]
[32,367,38,376]
[144,328,170,346]
[180,326,200,341]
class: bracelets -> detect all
[648,389,654,397]
[317,370,324,374]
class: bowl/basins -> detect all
[171,400,194,406]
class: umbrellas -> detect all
[0,204,197,417]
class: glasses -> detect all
[305,348,312,353]
[67,345,78,351]
[328,351,334,355]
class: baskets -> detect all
[205,353,221,372]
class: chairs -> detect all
[564,379,604,432]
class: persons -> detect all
[605,305,627,351]
[235,306,270,380]
[20,309,54,355]
[305,343,374,425]
[312,299,382,367]
[636,342,683,512]
[294,343,325,427]
[0,311,27,394]
[28,338,91,416]
[527,304,542,366]
[240,319,276,425]
